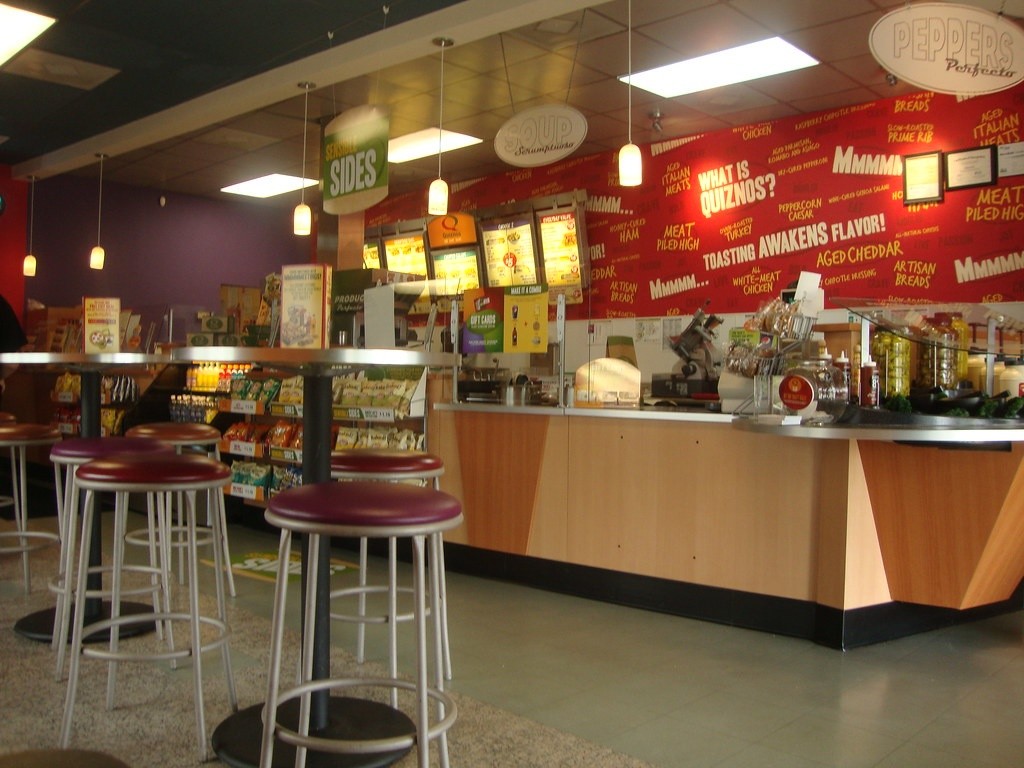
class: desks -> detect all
[0,353,171,644]
[171,346,463,768]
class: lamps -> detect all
[294,85,312,236]
[618,0,642,187]
[22,176,37,277]
[89,154,105,268]
[428,41,448,216]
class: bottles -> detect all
[168,394,219,424]
[851,345,861,401]
[186,361,250,393]
[863,356,878,409]
[818,347,833,400]
[835,351,851,401]
[981,357,1024,403]
[512,327,517,345]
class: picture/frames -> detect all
[903,150,944,205]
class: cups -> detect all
[512,306,518,318]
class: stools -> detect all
[0,422,63,596]
[259,481,463,768]
[125,423,237,598]
[330,446,452,682]
[0,411,22,546]
[49,437,178,683]
[58,453,238,764]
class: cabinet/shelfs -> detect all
[50,364,263,525]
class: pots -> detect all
[912,389,1010,414]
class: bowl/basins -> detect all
[510,236,520,244]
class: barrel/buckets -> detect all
[968,349,1024,401]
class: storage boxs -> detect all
[267,441,303,464]
[332,366,427,424]
[223,464,272,502]
[187,333,214,346]
[201,316,228,334]
[270,372,355,418]
[219,378,279,416]
[218,438,266,458]
[217,333,241,346]
